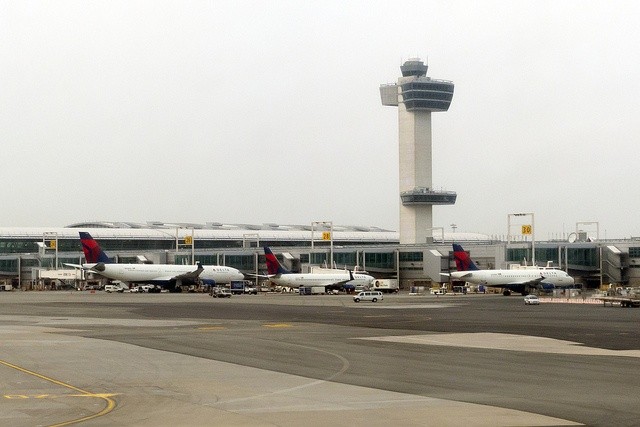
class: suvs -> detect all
[105,285,124,293]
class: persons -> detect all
[607,285,634,307]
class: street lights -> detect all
[312,221,319,249]
[186,227,194,265]
[176,227,182,252]
[323,221,333,269]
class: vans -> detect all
[353,292,379,302]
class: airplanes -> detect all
[247,247,376,295]
[438,244,574,296]
[63,232,245,293]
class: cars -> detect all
[524,295,539,305]
